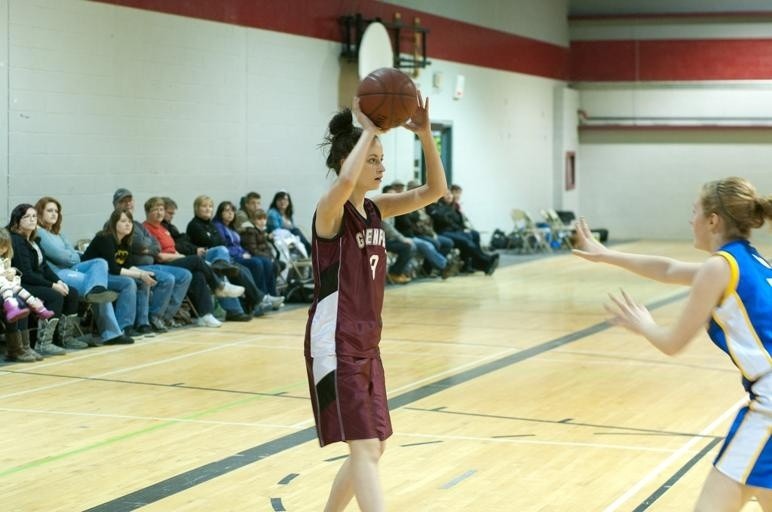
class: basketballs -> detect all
[360,68,420,130]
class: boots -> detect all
[4,298,30,323]
[58,314,90,348]
[4,327,43,362]
[34,318,67,354]
[473,246,501,275]
[30,298,55,321]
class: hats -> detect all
[391,180,406,188]
[114,188,132,202]
[407,180,421,190]
[254,210,267,218]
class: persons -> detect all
[0,189,315,364]
[380,180,502,286]
[570,175,771,512]
[303,89,448,511]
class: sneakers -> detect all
[216,282,245,298]
[439,258,473,280]
[85,288,119,304]
[387,271,412,284]
[229,313,252,321]
[103,318,183,345]
[210,261,239,276]
[253,280,304,317]
[197,313,222,328]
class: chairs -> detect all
[512,208,552,255]
[539,206,585,250]
